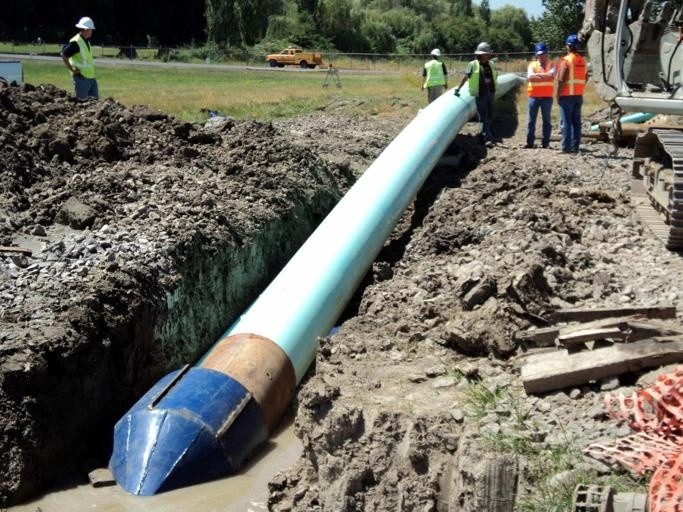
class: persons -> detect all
[554,35,588,155]
[454,41,498,147]
[62,16,99,100]
[522,43,557,150]
[421,48,448,103]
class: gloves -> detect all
[454,89,461,97]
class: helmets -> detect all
[566,35,579,46]
[75,17,95,29]
[431,49,441,57]
[535,43,548,55]
[475,42,493,55]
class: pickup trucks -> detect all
[264,44,322,70]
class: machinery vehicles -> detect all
[575,0,682,249]
